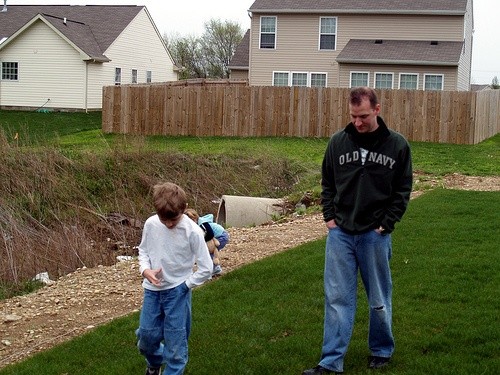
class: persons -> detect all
[182,208,230,276]
[302,86,414,375]
[137,182,214,375]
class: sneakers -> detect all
[303,366,343,375]
[367,356,389,369]
[212,264,221,276]
[145,364,161,375]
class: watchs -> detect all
[378,225,388,237]
